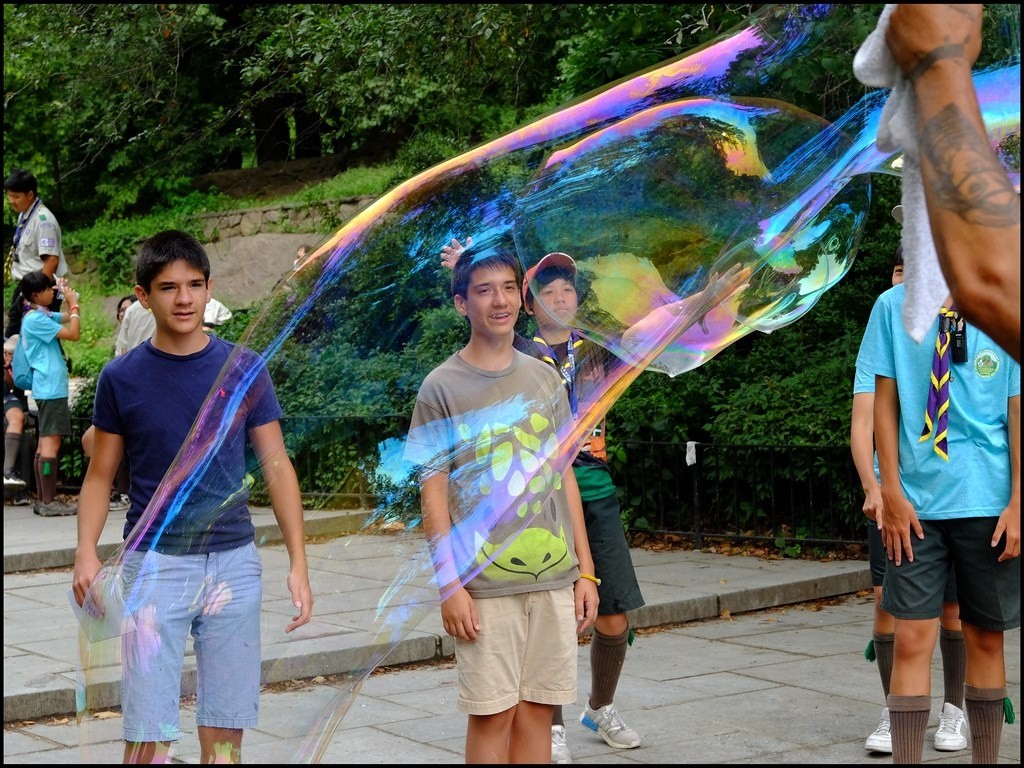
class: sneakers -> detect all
[934,702,967,751]
[3,472,26,485]
[865,708,892,752]
[551,725,572,764]
[33,499,77,517]
[14,492,31,506]
[578,692,641,749]
[109,494,130,510]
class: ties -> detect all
[4,219,27,283]
[919,304,956,463]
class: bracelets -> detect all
[580,574,601,586]
[70,314,80,319]
[69,304,79,313]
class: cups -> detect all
[56,285,80,302]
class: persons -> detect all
[72,229,314,765]
[2,171,317,515]
[402,241,599,764]
[441,236,750,766]
[850,2,1020,764]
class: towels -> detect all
[850,3,951,345]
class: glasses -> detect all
[118,307,127,313]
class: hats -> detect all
[522,252,577,302]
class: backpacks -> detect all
[12,307,36,390]
